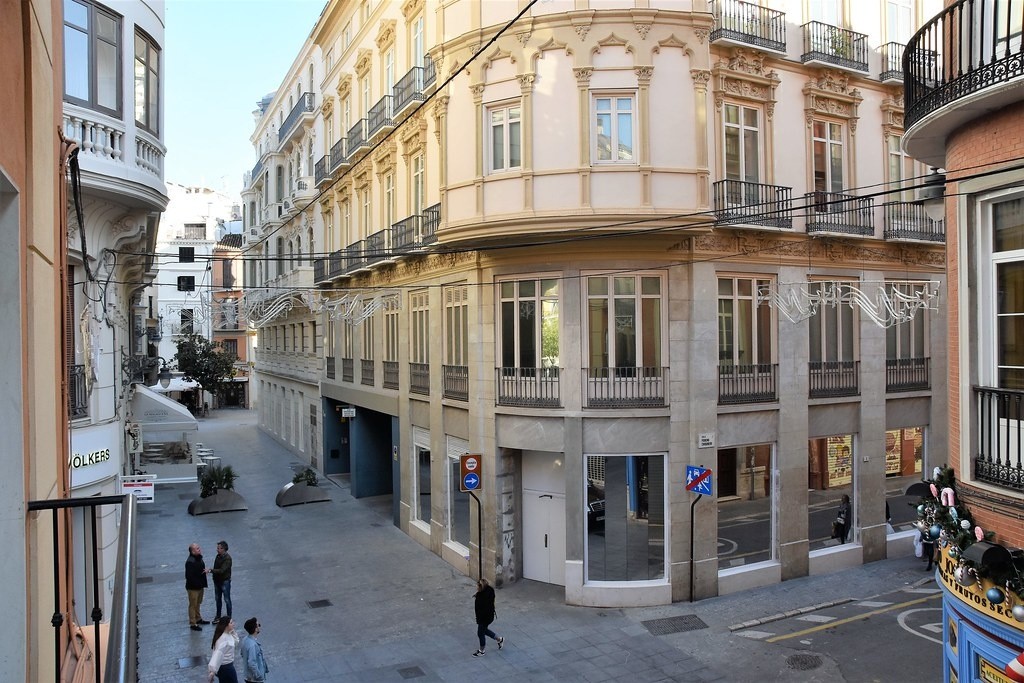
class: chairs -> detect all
[197,402,210,416]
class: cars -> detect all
[588,478,605,530]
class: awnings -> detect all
[218,377,249,382]
[158,379,203,388]
[132,387,198,431]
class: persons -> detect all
[241,617,269,683]
[207,615,240,683]
[836,495,851,544]
[203,541,233,623]
[185,543,209,631]
[472,579,505,657]
[919,532,939,570]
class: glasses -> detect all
[256,624,261,628]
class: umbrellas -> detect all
[148,383,192,397]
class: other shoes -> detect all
[935,562,938,565]
[190,625,202,631]
[212,618,221,624]
[197,619,210,624]
[926,565,932,571]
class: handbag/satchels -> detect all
[831,521,838,537]
[887,522,895,534]
[933,548,941,561]
[913,530,923,557]
[847,529,851,541]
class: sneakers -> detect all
[497,637,505,650]
[472,650,485,657]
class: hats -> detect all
[244,617,257,630]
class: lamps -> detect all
[912,164,946,221]
[121,344,173,398]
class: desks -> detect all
[196,442,203,453]
[197,453,214,470]
[148,457,167,463]
[143,453,163,458]
[196,462,207,481]
[197,449,214,468]
[201,456,222,473]
[149,444,164,449]
[144,449,163,453]
